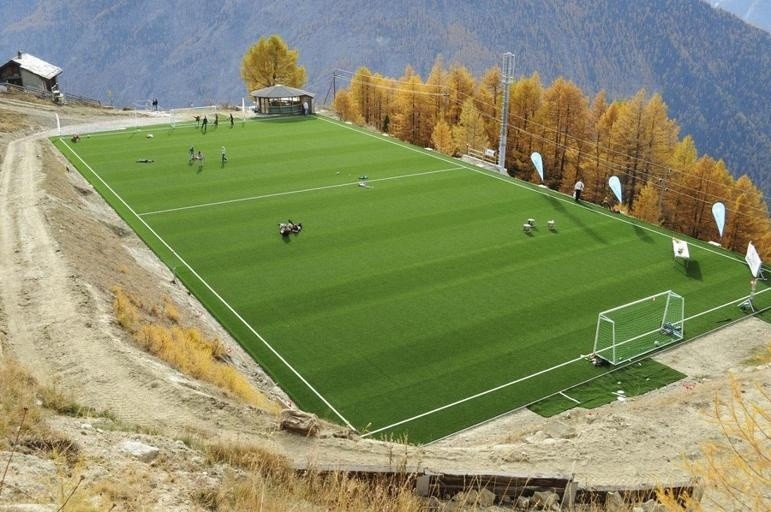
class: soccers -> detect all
[654,341,659,345]
[337,172,340,175]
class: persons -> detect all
[201,116,208,133]
[303,100,310,116]
[229,113,235,126]
[220,146,230,163]
[194,116,202,125]
[195,151,206,166]
[575,178,586,201]
[72,134,80,144]
[749,278,758,301]
[188,147,196,161]
[279,218,303,237]
[213,113,220,127]
